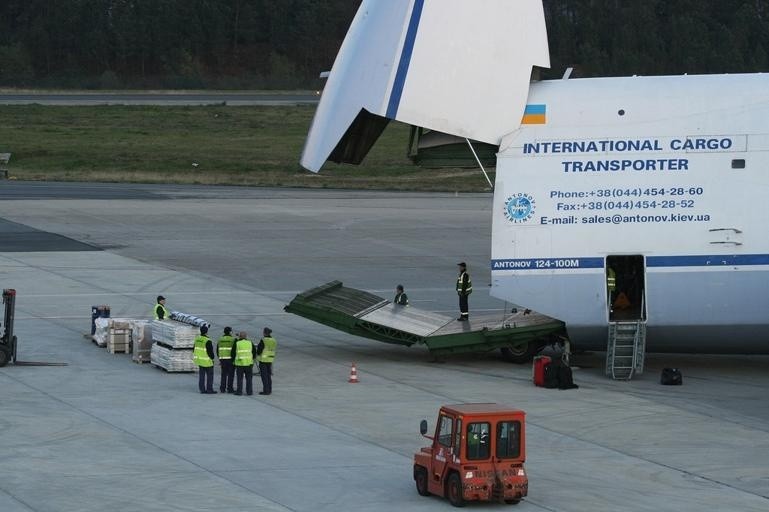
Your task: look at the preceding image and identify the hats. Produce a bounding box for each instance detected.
[157,296,165,301]
[457,262,466,267]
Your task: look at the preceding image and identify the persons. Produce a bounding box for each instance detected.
[153,296,167,321]
[193,325,277,395]
[394,285,408,305]
[608,268,616,291]
[537,337,570,361]
[455,262,472,321]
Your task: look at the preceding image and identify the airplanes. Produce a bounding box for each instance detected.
[282,0,768,380]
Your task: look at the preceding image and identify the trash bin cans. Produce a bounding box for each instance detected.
[91,305,109,347]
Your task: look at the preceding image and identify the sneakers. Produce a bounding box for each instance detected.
[456,315,468,320]
[200,390,272,396]
